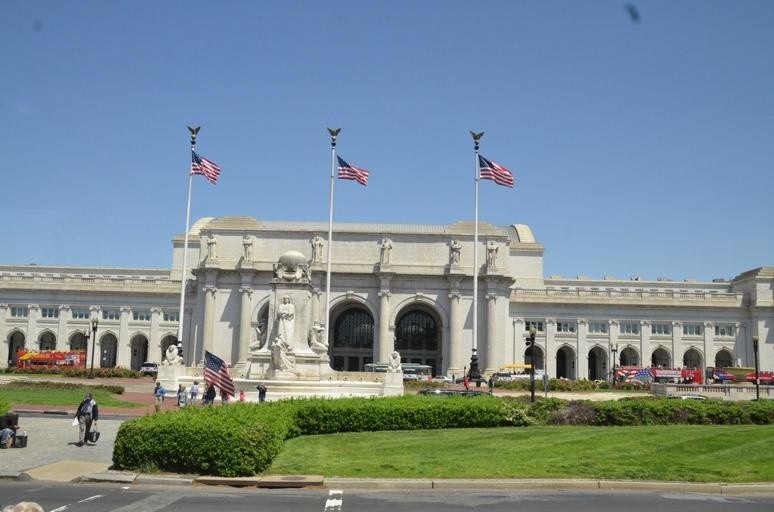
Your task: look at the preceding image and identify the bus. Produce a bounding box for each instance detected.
[364,361,433,383]
[14,347,88,375]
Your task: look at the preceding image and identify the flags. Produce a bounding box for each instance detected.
[477,152,513,188]
[203,351,234,397]
[615,366,655,382]
[336,154,369,186]
[190,148,220,187]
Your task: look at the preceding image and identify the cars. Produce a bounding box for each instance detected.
[432,374,453,384]
[454,376,467,384]
[591,379,646,386]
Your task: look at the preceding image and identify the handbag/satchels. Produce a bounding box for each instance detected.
[12,435,27,447]
[89,432,99,442]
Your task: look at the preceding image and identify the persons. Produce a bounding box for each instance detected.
[255,384,266,402]
[487,377,492,393]
[377,236,393,264]
[72,392,100,447]
[151,381,165,413]
[276,294,295,346]
[692,364,701,384]
[205,231,218,259]
[175,379,232,408]
[485,240,499,269]
[239,389,244,401]
[242,232,253,260]
[311,234,324,263]
[0,410,21,449]
[682,364,689,382]
[450,238,463,265]
[463,374,470,389]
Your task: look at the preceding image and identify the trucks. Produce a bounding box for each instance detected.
[613,363,738,389]
[743,371,774,386]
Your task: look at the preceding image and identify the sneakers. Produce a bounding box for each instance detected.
[0,444,7,449]
[79,440,83,446]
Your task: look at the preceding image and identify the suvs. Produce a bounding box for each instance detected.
[139,362,158,376]
[416,387,497,399]
[666,393,709,401]
[489,371,512,385]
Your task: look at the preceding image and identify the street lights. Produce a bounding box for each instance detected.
[525,321,537,404]
[751,334,762,400]
[608,342,620,383]
[87,316,99,379]
